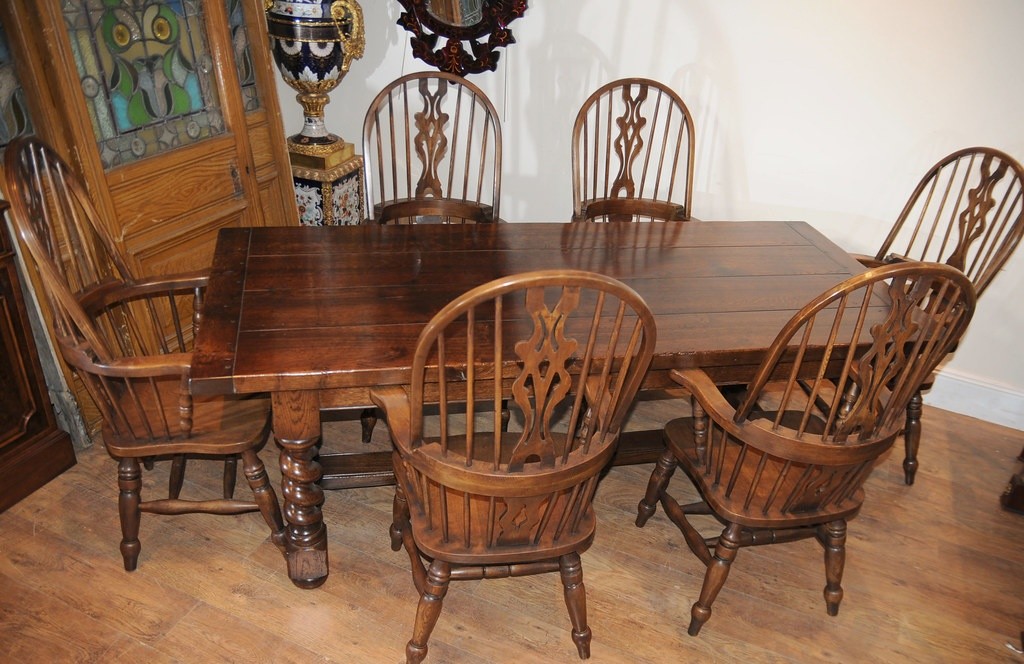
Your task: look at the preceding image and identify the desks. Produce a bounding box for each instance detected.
[190,222,957,592]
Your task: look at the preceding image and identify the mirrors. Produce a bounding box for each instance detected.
[396,0,528,84]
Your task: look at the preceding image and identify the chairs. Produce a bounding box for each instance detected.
[634,260,977,635]
[792,147,1024,482]
[369,267,657,663]
[360,73,504,225]
[2,135,288,572]
[572,77,702,223]
